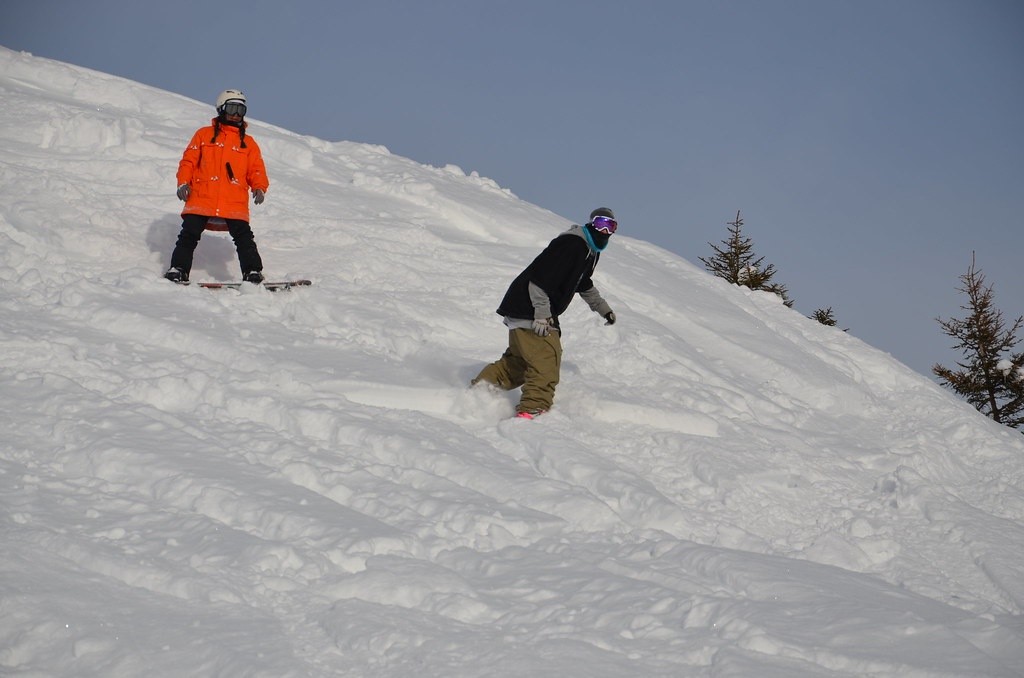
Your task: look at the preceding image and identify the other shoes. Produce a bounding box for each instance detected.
[517,411,541,420]
[243,272,263,284]
[166,264,189,283]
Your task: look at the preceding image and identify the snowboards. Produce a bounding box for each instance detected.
[172,278,314,293]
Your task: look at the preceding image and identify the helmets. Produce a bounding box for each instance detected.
[216,89,246,115]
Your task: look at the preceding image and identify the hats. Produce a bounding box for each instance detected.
[591,207,614,220]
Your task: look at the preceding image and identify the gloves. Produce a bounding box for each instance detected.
[604,311,616,326]
[177,183,192,201]
[532,316,554,337]
[253,189,265,205]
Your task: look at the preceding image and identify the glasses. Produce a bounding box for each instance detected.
[592,216,617,235]
[222,103,247,117]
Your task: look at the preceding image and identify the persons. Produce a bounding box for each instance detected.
[466,207,618,420]
[163,89,274,287]
